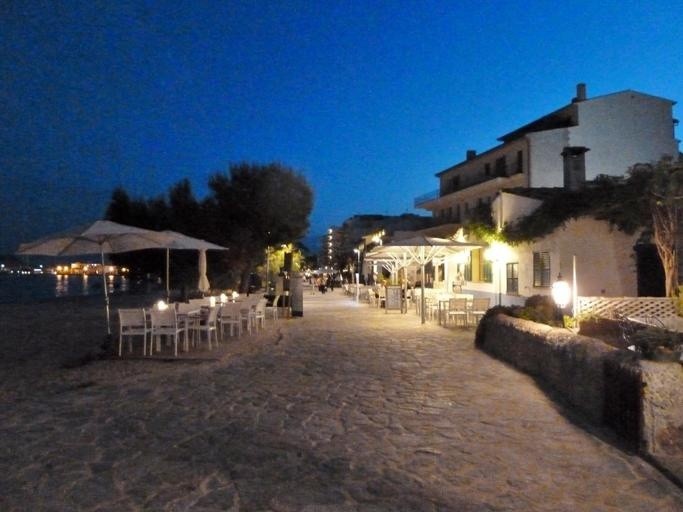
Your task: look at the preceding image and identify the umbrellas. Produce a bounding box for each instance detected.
[156,230,230,305]
[197,248,211,299]
[14,220,183,335]
[362,230,490,324]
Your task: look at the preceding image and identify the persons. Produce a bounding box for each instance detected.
[309,272,348,295]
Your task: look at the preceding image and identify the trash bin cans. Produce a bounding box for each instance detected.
[386,286,402,309]
[289,277,303,316]
[451,281,462,293]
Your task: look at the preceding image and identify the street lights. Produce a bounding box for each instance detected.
[352,248,363,305]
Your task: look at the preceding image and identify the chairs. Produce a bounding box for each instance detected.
[344,283,491,330]
[117,292,280,357]
